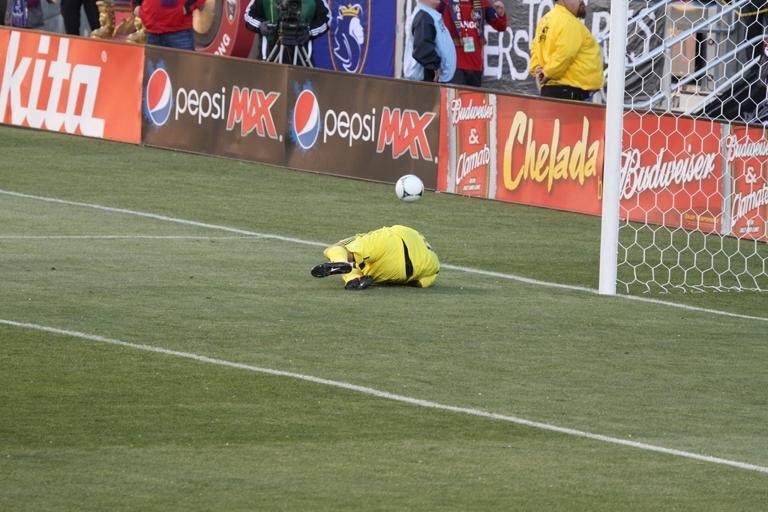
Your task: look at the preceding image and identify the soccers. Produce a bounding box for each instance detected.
[395,174,424,203]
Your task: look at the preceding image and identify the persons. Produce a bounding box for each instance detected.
[133,0,206,51]
[528,0,604,101]
[311,224,440,289]
[244,0,333,68]
[403,0,457,84]
[47,0,101,36]
[436,0,507,87]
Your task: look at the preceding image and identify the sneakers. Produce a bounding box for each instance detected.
[311,262,353,277]
[345,274,375,290]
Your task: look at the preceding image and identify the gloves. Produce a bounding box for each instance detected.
[260,20,272,35]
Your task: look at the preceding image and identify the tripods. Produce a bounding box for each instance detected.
[265,36,314,68]
